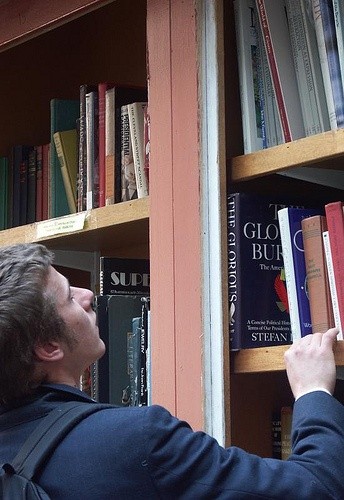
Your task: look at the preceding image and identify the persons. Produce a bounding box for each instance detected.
[0,242,343,500]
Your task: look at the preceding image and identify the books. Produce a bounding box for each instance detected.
[225,188,344,351]
[271,405,295,460]
[1,82,151,229]
[231,0,344,154]
[76,256,151,407]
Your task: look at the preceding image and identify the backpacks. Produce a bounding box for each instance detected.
[0,401,118,500]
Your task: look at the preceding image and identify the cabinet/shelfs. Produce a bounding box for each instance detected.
[0,0,176,420]
[169,0,344,456]
